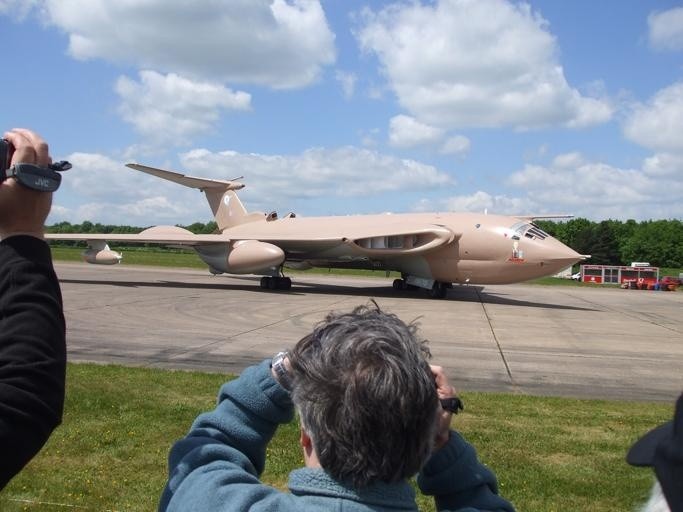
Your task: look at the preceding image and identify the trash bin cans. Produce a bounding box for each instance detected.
[630,280,636,289]
[654,283,659,290]
[642,284,648,290]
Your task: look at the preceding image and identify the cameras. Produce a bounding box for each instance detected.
[0,139,16,184]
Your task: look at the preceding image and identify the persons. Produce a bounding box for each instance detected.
[156,294,514,510]
[0,124,66,497]
[625,394,682,512]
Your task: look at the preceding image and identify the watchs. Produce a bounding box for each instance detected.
[270,348,295,393]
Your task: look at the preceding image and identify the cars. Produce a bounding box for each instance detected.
[571,272,581,281]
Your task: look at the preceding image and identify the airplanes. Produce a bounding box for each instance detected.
[43,162,592,299]
